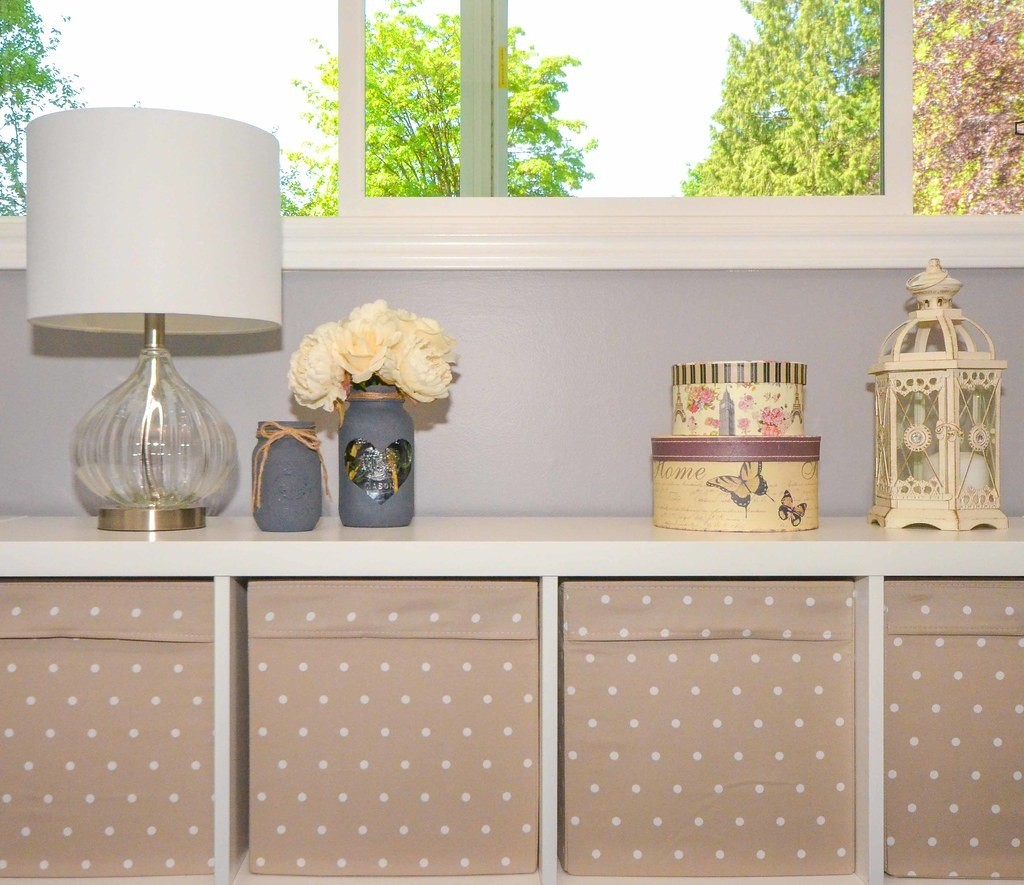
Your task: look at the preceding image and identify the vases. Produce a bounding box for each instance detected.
[335,379,416,528]
[251,421,331,532]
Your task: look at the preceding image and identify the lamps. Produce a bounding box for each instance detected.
[25,108,287,533]
[869,257,1005,535]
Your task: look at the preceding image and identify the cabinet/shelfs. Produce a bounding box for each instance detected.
[0,514,1024,884]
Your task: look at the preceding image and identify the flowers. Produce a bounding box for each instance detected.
[287,299,458,414]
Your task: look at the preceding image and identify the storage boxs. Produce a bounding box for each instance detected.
[651,434,822,532]
[671,358,806,436]
